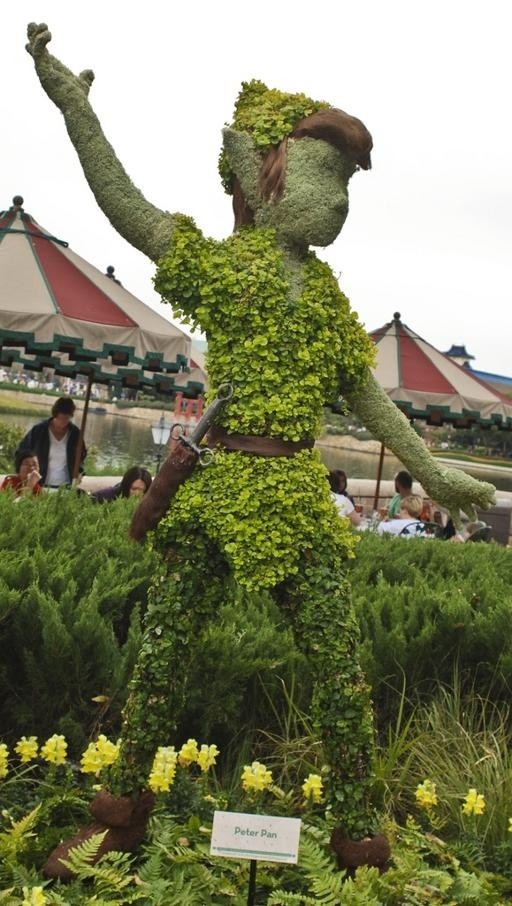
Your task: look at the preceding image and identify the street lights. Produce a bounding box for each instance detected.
[150,411,173,474]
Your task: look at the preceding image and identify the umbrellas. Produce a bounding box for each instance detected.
[0,266,209,489]
[323,312,512,509]
[0,196,192,373]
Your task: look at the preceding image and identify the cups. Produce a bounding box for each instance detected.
[381,508,388,523]
[354,504,365,512]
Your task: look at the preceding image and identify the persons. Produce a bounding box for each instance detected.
[20,398,87,490]
[90,467,152,505]
[0,449,43,497]
[325,469,478,539]
[25,22,495,886]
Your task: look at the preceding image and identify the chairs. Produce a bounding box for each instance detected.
[398,517,492,543]
